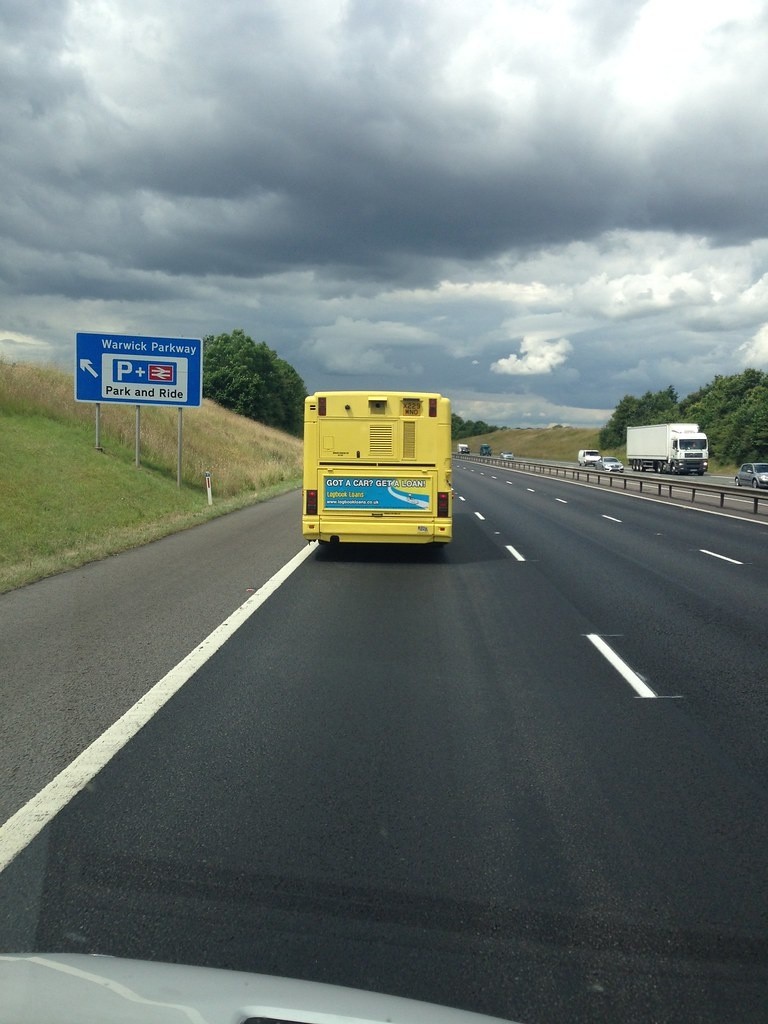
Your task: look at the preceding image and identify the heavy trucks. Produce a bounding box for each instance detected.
[626,422,709,477]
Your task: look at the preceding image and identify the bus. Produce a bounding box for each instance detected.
[300,390,453,552]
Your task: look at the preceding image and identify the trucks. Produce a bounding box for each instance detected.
[481,444,492,456]
[578,449,602,466]
[457,444,470,454]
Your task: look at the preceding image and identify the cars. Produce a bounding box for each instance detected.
[500,451,515,461]
[734,461,768,488]
[594,455,625,474]
